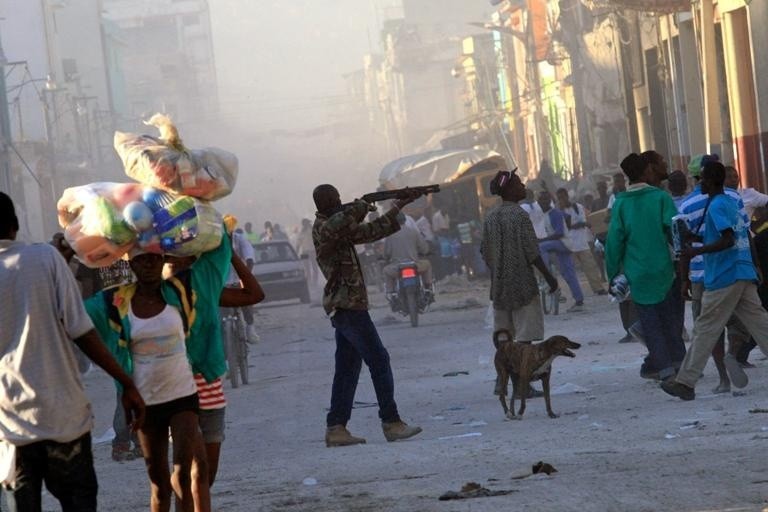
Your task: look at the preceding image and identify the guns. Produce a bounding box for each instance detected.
[359,183,441,221]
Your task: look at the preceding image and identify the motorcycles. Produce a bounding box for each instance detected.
[386,261,434,328]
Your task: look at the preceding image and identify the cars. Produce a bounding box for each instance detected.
[246,243,310,305]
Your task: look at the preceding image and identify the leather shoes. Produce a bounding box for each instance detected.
[640,367,658,378]
[660,380,695,400]
[325,425,366,448]
[619,334,637,343]
[382,422,422,441]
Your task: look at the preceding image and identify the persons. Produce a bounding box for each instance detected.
[357,205,477,302]
[479,168,558,399]
[619,150,767,401]
[313,183,424,448]
[0,191,148,512]
[243,216,319,282]
[47,212,266,512]
[521,172,626,310]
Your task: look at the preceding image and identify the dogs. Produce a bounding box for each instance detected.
[491,327,583,421]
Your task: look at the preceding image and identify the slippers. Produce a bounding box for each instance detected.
[712,386,729,394]
[722,353,749,388]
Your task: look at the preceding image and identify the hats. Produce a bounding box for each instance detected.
[687,153,719,179]
[489,166,518,196]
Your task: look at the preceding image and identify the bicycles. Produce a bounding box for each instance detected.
[220,306,253,389]
[539,257,566,317]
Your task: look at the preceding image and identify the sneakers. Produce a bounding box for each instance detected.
[566,304,584,312]
[246,329,261,344]
